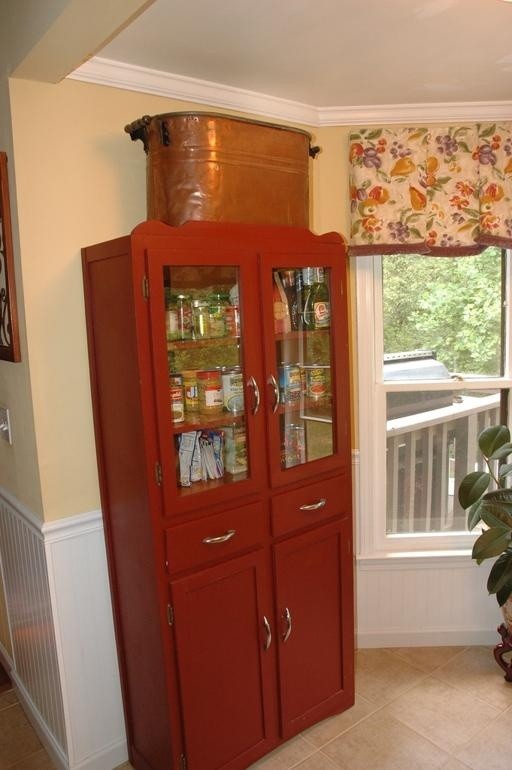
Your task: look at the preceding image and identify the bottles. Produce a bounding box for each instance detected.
[163,291,241,343]
[169,368,224,423]
[273,267,332,331]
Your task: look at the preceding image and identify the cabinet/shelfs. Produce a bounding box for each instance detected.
[81,219,355,770]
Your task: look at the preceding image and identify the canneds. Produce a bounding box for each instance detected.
[163,292,241,342]
[278,360,327,405]
[167,364,245,424]
[279,423,307,469]
